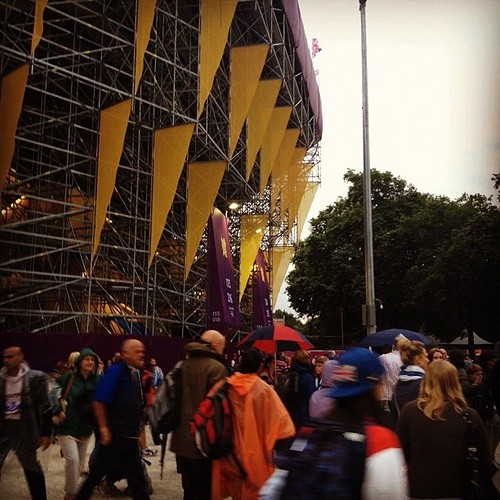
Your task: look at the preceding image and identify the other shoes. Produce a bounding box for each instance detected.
[79,470,89,478]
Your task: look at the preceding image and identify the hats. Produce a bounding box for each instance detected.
[322,348,383,397]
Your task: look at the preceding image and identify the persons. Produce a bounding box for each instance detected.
[48,341,499,434]
[49,346,106,499]
[162,329,232,500]
[255,346,412,500]
[0,346,55,500]
[393,359,491,500]
[72,338,155,499]
[190,346,297,499]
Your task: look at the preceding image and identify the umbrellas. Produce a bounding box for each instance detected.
[356,328,434,349]
[236,325,316,354]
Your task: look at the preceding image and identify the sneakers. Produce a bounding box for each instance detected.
[139,447,157,456]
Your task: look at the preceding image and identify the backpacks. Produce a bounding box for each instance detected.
[272,416,376,500]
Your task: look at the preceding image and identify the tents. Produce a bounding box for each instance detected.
[448,328,495,359]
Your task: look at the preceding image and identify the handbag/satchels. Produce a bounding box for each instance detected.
[61,374,74,412]
[194,379,233,459]
[463,410,490,500]
[155,368,183,435]
